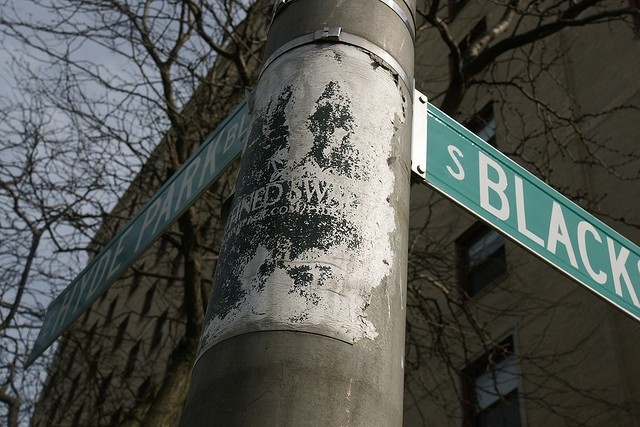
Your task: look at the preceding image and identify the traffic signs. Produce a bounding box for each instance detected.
[21,82,251,372]
[412,87,640,322]
